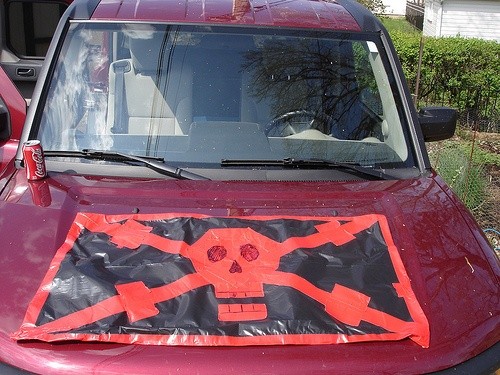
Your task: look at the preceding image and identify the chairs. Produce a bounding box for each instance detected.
[104,31,195,135]
[237,38,326,137]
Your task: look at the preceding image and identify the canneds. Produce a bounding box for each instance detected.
[21,139,48,181]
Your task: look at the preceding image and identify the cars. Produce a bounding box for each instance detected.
[0,0,500,375]
